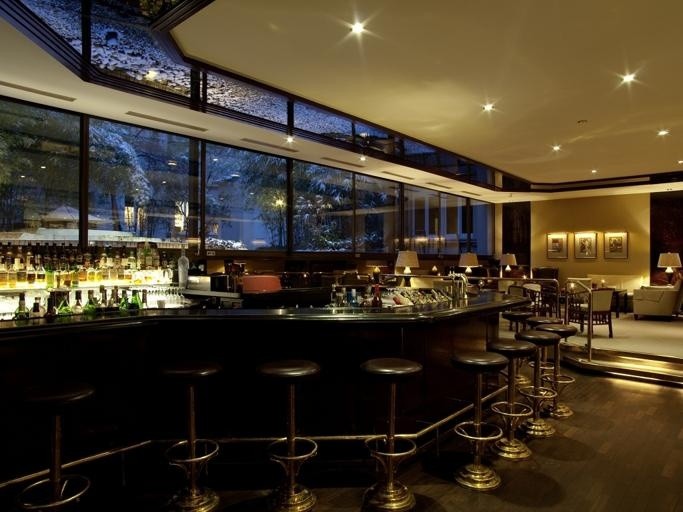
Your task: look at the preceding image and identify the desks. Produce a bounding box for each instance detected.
[542,289,589,333]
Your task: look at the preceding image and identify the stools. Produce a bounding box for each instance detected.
[502,311,534,386]
[453,352,509,491]
[515,330,560,439]
[526,317,561,386]
[163,363,222,511]
[19,386,94,511]
[487,340,537,461]
[512,312,533,361]
[362,357,423,511]
[259,362,321,511]
[536,324,577,418]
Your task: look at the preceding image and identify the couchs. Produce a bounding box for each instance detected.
[673,287,683,317]
[587,274,643,313]
[568,278,591,312]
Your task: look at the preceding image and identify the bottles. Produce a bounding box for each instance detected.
[349,288,357,312]
[34,243,41,266]
[15,300,28,319]
[51,242,176,290]
[109,289,117,307]
[57,293,70,314]
[118,290,129,316]
[26,257,35,289]
[19,292,26,301]
[141,290,149,308]
[36,259,46,288]
[113,285,120,299]
[46,262,54,288]
[16,259,26,289]
[4,241,14,262]
[14,245,25,270]
[24,240,33,269]
[0,242,4,257]
[99,285,104,294]
[83,290,94,309]
[0,258,7,289]
[129,289,139,316]
[371,284,382,310]
[71,291,82,313]
[30,302,40,316]
[28,297,44,316]
[137,291,141,310]
[177,245,189,288]
[97,290,108,308]
[43,242,49,267]
[44,298,55,317]
[7,257,16,288]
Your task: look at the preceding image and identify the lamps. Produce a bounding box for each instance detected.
[657,252,682,287]
[396,250,420,287]
[459,253,478,276]
[366,260,388,284]
[500,254,517,277]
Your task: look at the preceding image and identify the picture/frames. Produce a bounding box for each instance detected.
[574,231,597,259]
[547,232,568,259]
[604,232,628,259]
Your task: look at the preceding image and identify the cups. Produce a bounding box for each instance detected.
[146,287,186,307]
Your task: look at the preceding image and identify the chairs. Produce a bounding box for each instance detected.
[532,268,559,317]
[503,285,525,331]
[530,279,559,317]
[498,279,515,292]
[565,288,616,362]
[634,280,681,321]
